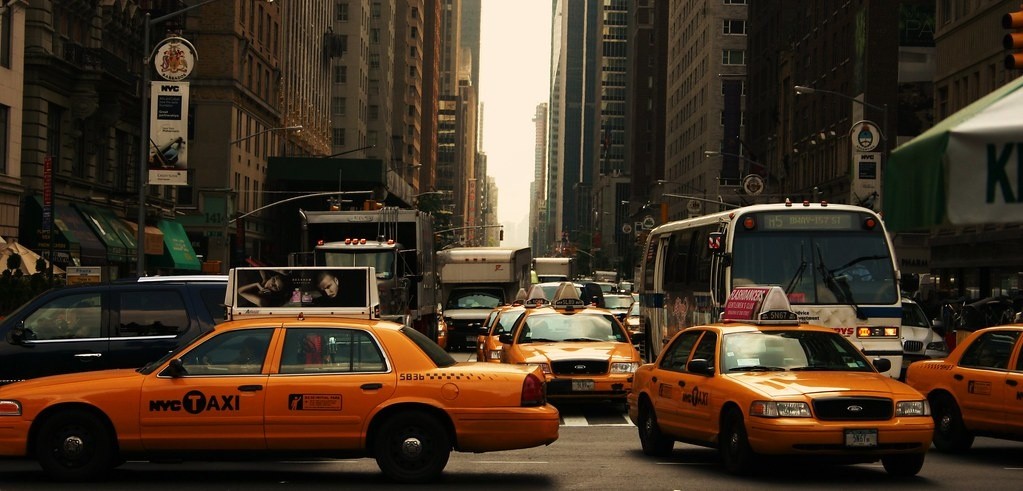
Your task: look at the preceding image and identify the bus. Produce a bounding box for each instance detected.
[637,198,918,380]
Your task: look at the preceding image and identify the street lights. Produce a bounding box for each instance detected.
[704,151,782,203]
[795,85,888,173]
[223,124,304,276]
[659,180,706,215]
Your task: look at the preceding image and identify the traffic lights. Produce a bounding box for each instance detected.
[1001,5,1023,75]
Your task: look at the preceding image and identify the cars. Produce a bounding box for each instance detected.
[906,323,1023,458]
[499,281,647,414]
[901,297,949,379]
[623,301,641,345]
[626,285,937,479]
[438,303,452,350]
[525,277,639,302]
[602,294,635,324]
[0,266,560,491]
[476,288,526,362]
[483,284,552,363]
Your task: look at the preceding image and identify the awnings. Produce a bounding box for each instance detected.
[18,197,201,271]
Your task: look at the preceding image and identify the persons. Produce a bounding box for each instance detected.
[206,336,264,374]
[237,269,349,307]
[43,303,96,338]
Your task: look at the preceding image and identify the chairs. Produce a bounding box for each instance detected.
[727,350,784,368]
[531,321,550,339]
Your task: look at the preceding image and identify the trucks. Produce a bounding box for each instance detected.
[437,247,532,352]
[299,207,438,343]
[531,256,572,282]
